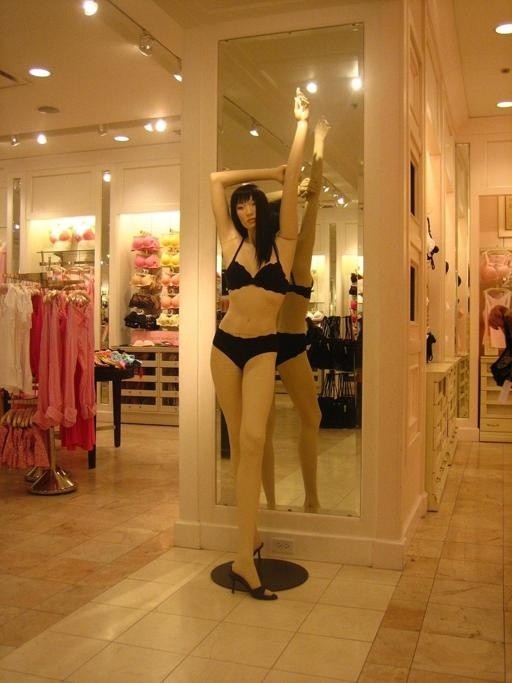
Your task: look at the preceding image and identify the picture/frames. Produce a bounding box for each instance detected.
[494,192,512,241]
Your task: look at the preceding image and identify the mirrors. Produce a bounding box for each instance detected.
[453,137,473,420]
[205,18,382,528]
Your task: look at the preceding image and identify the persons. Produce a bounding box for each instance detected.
[205,85,312,602]
[260,114,334,513]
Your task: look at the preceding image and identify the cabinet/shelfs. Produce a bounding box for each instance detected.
[271,258,327,391]
[476,351,511,444]
[424,348,469,516]
[111,345,182,428]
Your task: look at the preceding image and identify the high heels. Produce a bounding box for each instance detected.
[253,542,264,563]
[232,572,278,602]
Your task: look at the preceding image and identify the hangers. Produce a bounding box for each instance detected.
[481,242,510,258]
[2,265,92,310]
[131,225,179,317]
[0,392,42,433]
[481,282,510,299]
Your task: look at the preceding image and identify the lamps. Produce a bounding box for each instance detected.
[6,114,172,146]
[135,27,182,83]
[243,113,355,212]
